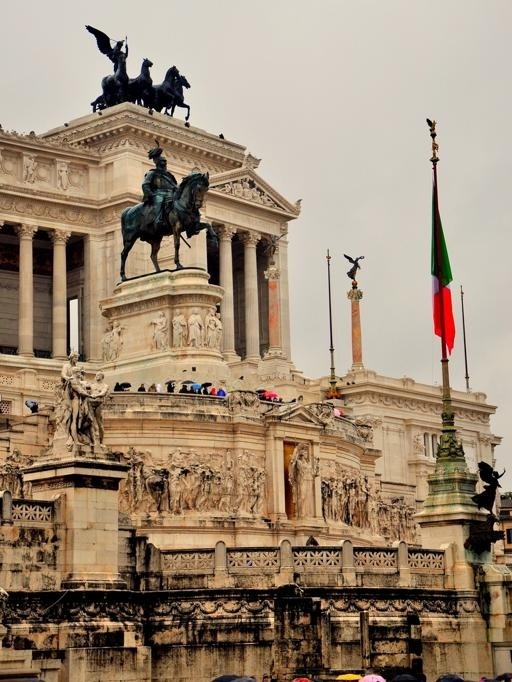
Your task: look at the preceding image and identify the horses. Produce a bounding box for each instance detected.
[100,52,189,120]
[118,171,218,281]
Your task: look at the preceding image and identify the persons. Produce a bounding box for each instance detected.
[150,307,224,351]
[59,162,71,190]
[26,155,35,182]
[296,450,319,517]
[471,461,506,514]
[142,139,177,230]
[85,24,128,72]
[115,379,229,396]
[61,350,107,446]
[347,257,361,282]
[259,392,296,403]
[30,402,38,413]
[108,320,123,359]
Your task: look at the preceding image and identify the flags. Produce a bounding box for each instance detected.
[430,166,455,353]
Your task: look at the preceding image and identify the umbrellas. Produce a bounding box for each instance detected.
[212,673,511,682]
[256,389,266,393]
